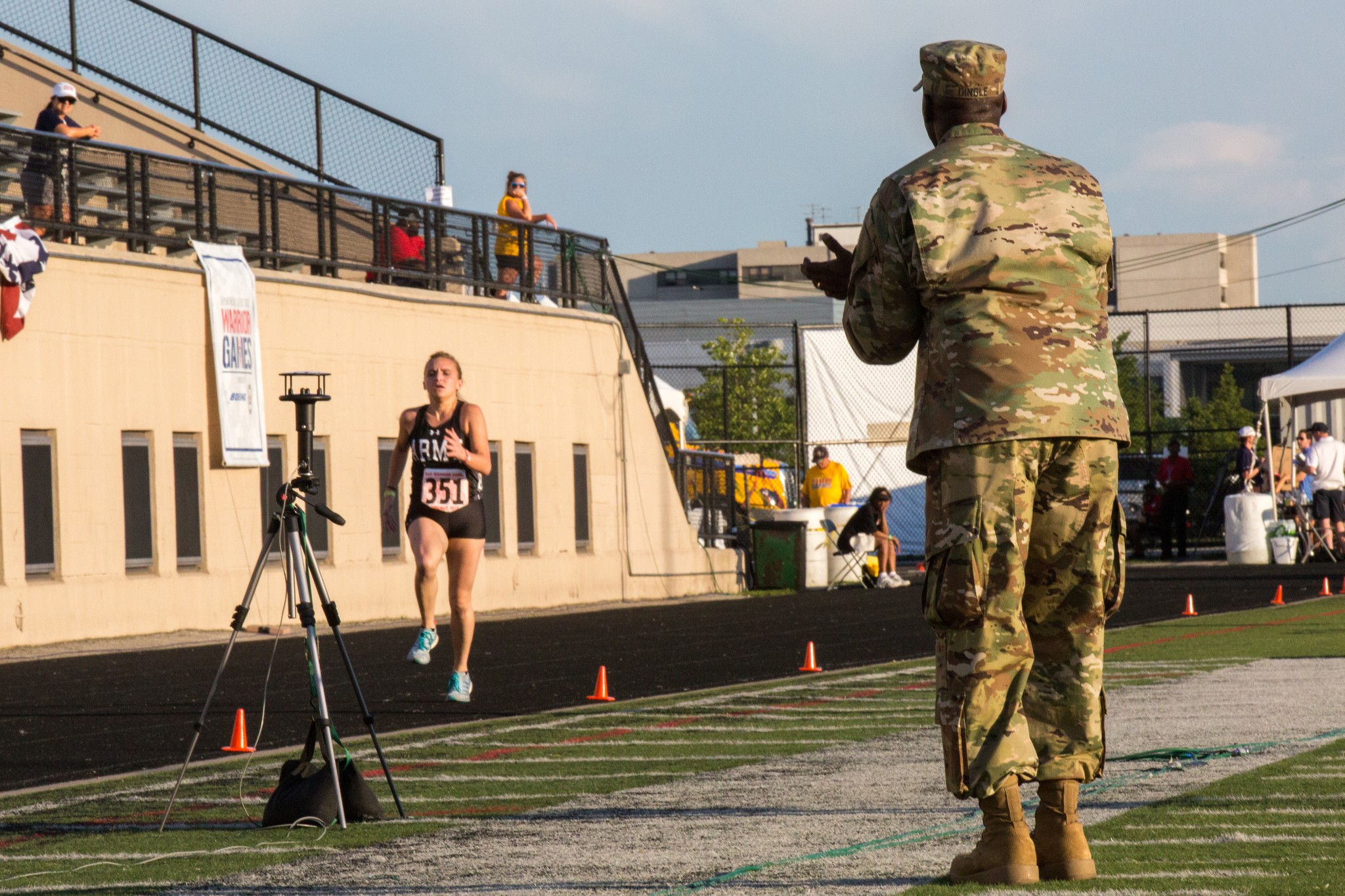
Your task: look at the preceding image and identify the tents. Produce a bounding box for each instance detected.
[1260,330,1345,523]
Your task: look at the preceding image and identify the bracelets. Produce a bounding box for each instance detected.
[384,492,398,498]
[463,448,472,463]
[889,534,892,540]
[522,196,527,199]
[385,486,398,492]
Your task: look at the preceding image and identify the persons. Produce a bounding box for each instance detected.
[1136,437,1192,561]
[837,486,910,589]
[800,37,1135,887]
[363,207,434,287]
[381,351,492,703]
[1284,423,1344,559]
[493,172,558,307]
[1236,427,1268,492]
[802,446,853,508]
[20,81,100,245]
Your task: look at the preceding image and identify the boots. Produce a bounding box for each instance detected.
[1031,780,1099,880]
[949,774,1041,884]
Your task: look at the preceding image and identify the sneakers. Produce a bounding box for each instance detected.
[447,669,473,702]
[407,619,440,665]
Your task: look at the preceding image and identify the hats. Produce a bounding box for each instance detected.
[913,40,1007,97]
[52,82,77,100]
[1307,422,1328,431]
[1238,425,1261,438]
[812,446,828,463]
[398,207,423,222]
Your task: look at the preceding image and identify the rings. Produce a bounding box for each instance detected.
[817,282,821,287]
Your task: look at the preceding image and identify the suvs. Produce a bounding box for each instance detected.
[1114,453,1165,548]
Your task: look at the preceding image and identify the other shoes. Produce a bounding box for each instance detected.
[1177,550,1187,558]
[1161,550,1171,559]
[877,574,911,589]
[524,296,541,305]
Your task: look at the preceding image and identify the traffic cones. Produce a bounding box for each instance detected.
[798,642,821,671]
[220,708,256,753]
[1340,576,1345,594]
[1271,584,1285,604]
[587,666,615,701]
[1319,577,1333,596]
[1182,593,1197,616]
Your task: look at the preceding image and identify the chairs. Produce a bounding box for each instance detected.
[820,519,877,592]
[1284,491,1338,566]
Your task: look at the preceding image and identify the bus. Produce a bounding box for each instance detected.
[652,409,797,538]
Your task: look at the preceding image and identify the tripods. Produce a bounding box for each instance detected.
[155,483,405,833]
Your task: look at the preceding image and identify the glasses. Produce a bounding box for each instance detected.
[1296,437,1304,440]
[57,97,76,104]
[511,182,525,188]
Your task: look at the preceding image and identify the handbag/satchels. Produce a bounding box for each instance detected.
[335,756,385,823]
[261,758,341,827]
